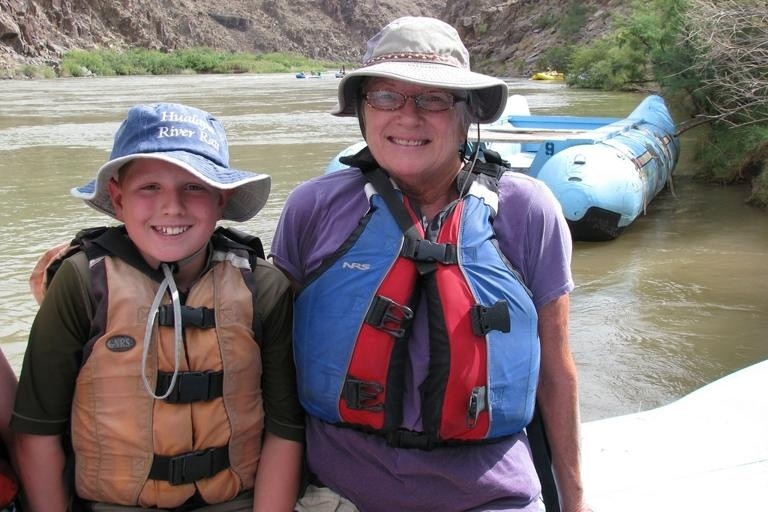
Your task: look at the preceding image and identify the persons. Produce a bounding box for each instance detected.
[29,15,594,512]
[7,103,306,512]
[0,348,27,512]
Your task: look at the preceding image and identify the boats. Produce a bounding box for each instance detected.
[323,93,681,244]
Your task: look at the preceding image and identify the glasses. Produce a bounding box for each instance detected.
[362,89,466,111]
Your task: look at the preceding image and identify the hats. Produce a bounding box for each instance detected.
[330,15,508,124]
[70,102,271,222]
